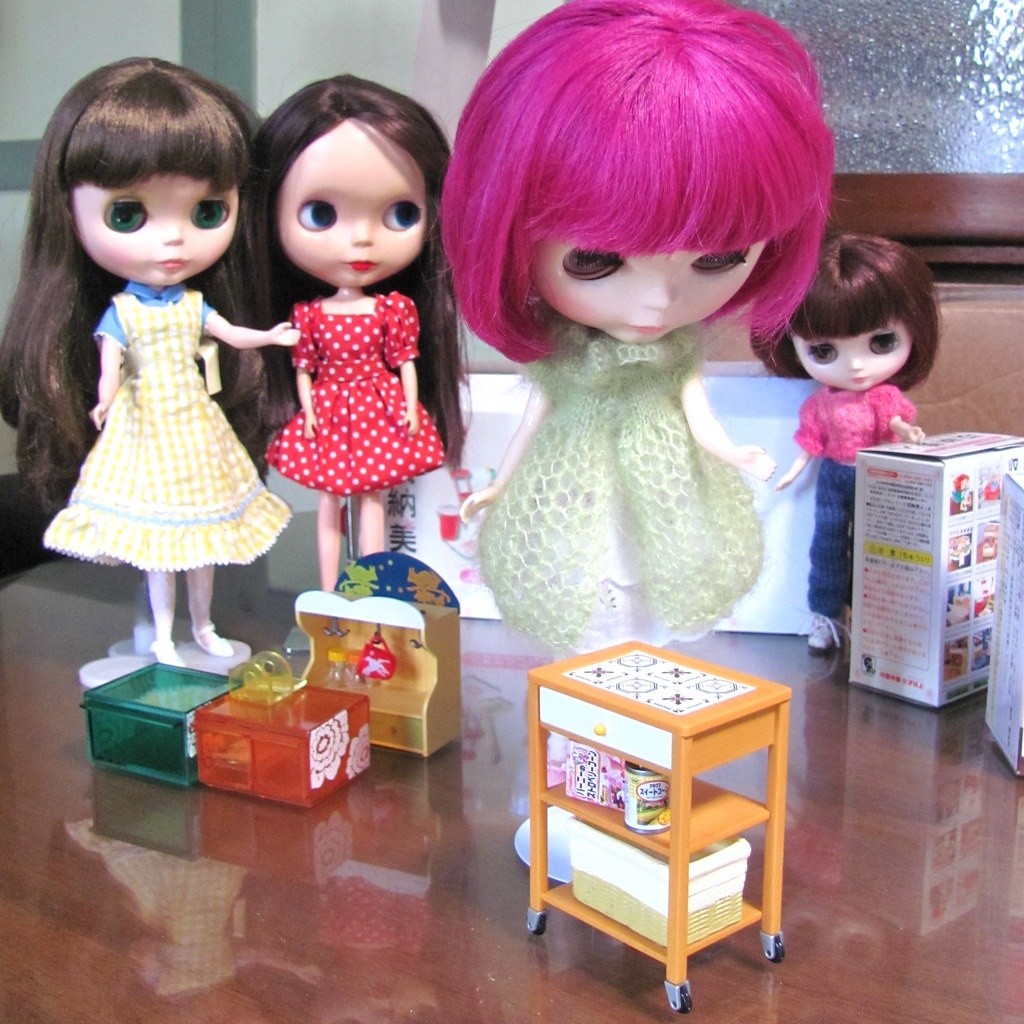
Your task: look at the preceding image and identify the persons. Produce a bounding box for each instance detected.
[445,0,834,678]
[241,74,465,596]
[0,55,299,665]
[748,232,941,650]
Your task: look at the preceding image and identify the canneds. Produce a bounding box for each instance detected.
[625,760,672,834]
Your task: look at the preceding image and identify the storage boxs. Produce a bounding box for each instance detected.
[846,433,1024,707]
[383,361,823,635]
[981,468,1024,778]
[81,663,244,787]
[569,816,753,948]
[191,679,371,808]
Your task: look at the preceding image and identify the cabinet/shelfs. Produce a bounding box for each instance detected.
[293,589,461,759]
[527,638,793,1012]
